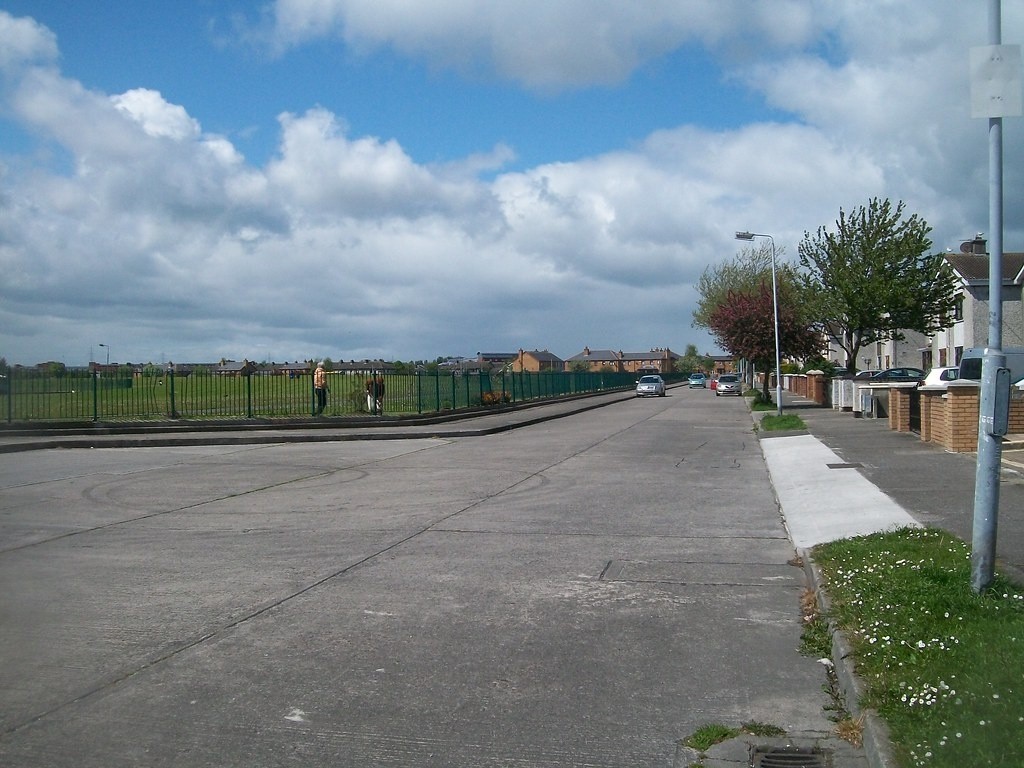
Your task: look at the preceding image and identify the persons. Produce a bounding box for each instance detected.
[365,377,385,411]
[314,362,330,415]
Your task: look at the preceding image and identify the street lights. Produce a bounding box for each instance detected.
[98,344,110,376]
[734,231,783,417]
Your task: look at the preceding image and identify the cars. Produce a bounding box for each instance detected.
[714,375,744,397]
[873,367,927,382]
[636,376,666,397]
[921,367,961,386]
[856,370,913,378]
[688,373,707,389]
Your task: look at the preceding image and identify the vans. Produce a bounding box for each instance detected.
[957,347,1024,385]
